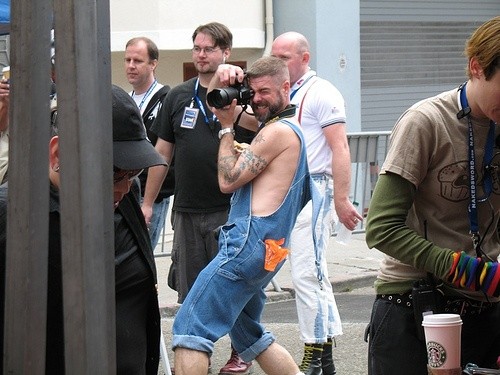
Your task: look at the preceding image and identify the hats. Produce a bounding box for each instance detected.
[51,84,168,170]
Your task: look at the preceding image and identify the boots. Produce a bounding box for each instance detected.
[299,342,324,375]
[322,337,336,375]
[218,343,256,375]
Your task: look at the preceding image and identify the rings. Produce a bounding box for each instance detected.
[354,218,357,221]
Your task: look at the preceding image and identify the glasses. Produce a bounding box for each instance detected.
[113,166,145,182]
[190,46,223,55]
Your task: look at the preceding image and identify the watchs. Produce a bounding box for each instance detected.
[219,128,236,139]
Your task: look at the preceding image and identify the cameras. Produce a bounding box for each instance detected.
[206,73,255,109]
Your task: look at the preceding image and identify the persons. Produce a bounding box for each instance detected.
[141,21,254,375]
[365,16,500,375]
[271,31,363,375]
[125,37,171,252]
[172,55,305,375]
[0,30,169,375]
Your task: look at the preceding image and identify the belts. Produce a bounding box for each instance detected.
[377,290,500,322]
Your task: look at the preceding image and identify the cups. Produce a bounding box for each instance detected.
[2,65,12,85]
[421,314,464,369]
